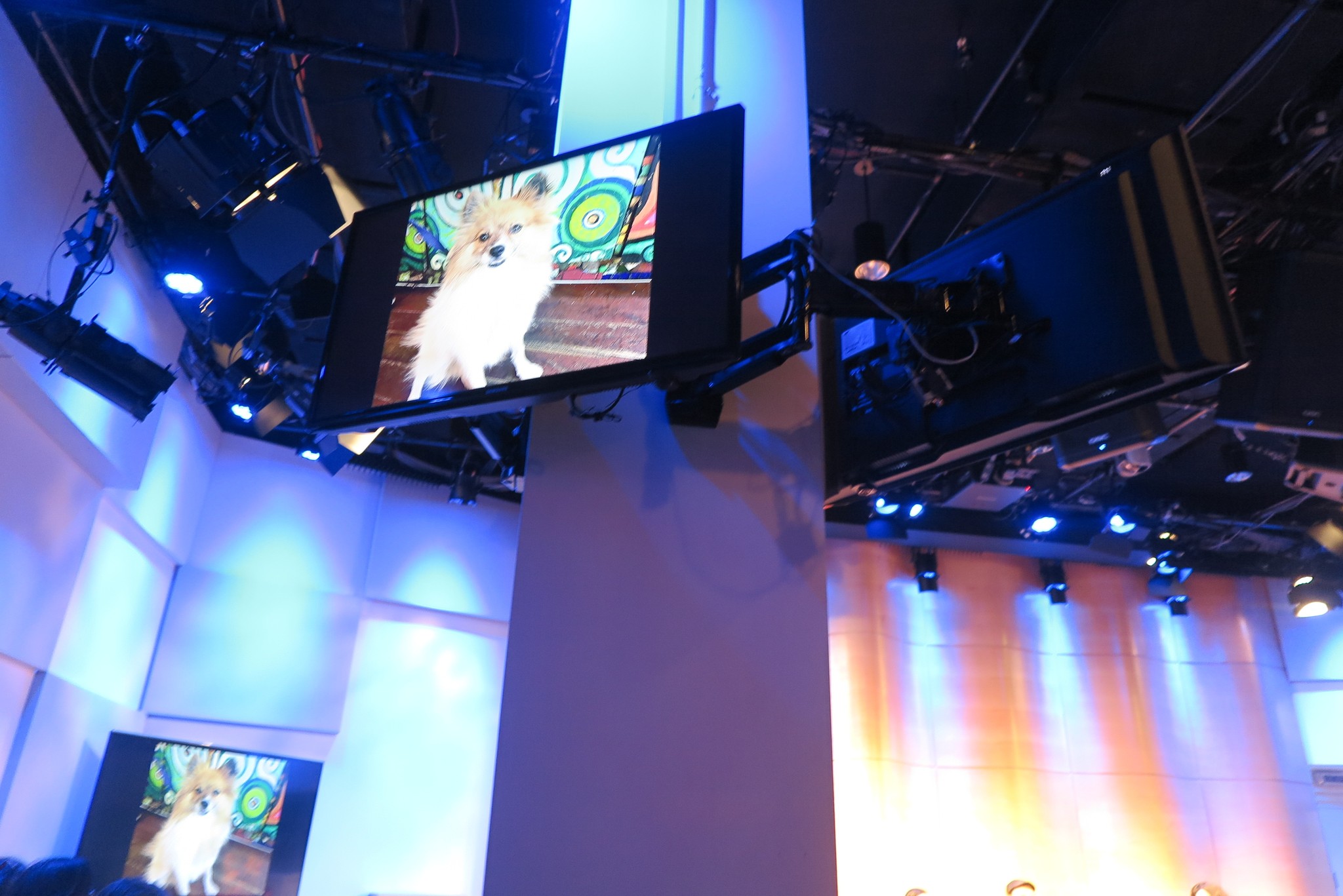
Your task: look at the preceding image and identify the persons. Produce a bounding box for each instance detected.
[0,854,167,896]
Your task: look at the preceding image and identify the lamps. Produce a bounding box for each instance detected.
[872,481,1329,618]
[86,15,482,508]
[850,159,891,281]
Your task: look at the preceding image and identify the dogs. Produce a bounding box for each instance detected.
[401,173,562,403]
[139,752,241,895]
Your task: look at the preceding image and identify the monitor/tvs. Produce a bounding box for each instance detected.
[820,120,1252,513]
[309,104,743,436]
[72,730,323,896]
